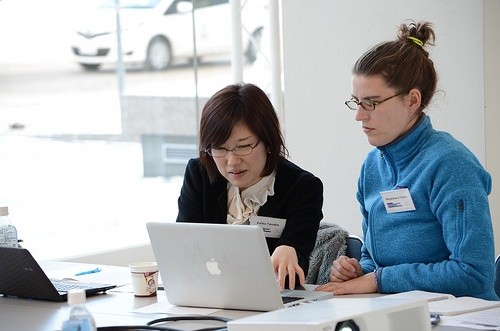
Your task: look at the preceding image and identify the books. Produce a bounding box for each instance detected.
[375,290,500,315]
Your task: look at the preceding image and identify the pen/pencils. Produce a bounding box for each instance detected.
[74,268,103,277]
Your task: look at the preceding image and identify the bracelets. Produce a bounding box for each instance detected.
[374,269,380,293]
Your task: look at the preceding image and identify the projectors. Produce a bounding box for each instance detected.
[227,293,434,331]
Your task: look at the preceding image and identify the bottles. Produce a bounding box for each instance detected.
[57,288,98,331]
[0,206,19,248]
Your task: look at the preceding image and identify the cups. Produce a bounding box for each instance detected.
[128,262,159,297]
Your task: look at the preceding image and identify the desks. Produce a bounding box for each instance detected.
[0,260,500,331]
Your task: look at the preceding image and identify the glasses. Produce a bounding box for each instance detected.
[344,87,413,111]
[204,138,261,158]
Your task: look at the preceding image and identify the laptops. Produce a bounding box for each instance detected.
[146,221,334,313]
[0,248,117,300]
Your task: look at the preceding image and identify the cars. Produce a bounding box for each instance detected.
[68,0,270,75]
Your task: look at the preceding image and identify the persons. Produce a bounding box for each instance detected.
[176,83,324,292]
[315,19,500,301]
